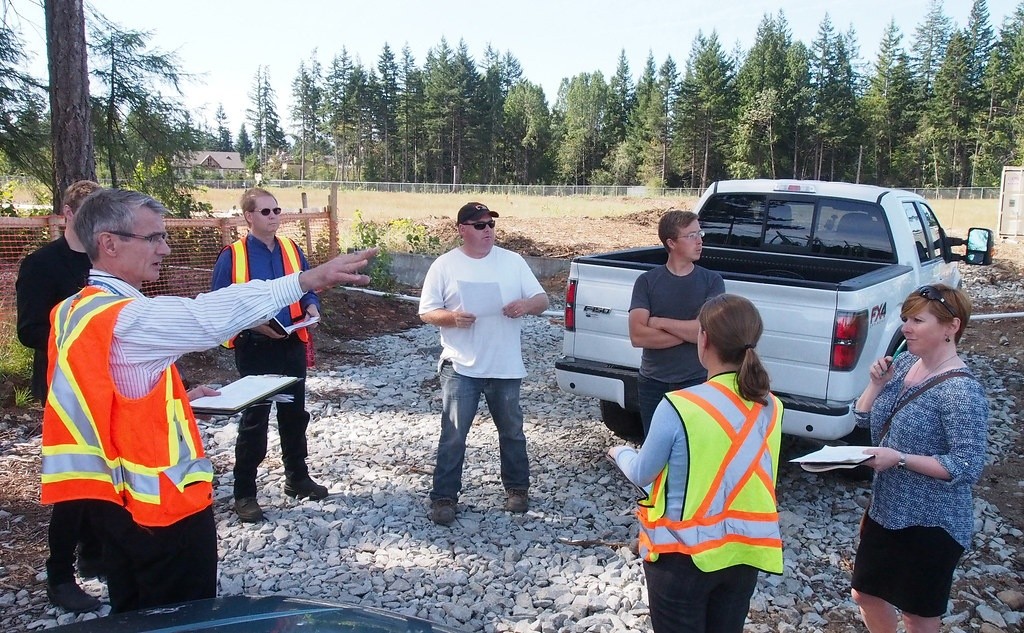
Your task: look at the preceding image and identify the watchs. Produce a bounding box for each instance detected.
[896,451,906,471]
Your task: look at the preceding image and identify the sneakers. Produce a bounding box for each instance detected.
[508,487,529,513]
[284,470,328,500]
[234,492,263,523]
[430,496,458,524]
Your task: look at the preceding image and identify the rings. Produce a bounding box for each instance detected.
[515,311,518,314]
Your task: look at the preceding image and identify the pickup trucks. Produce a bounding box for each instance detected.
[555,178,993,443]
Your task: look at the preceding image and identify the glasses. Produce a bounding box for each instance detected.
[248,207,282,216]
[461,219,496,231]
[919,285,958,320]
[96,230,169,247]
[674,229,705,240]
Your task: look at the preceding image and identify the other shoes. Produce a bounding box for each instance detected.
[77,563,107,577]
[46,577,102,612]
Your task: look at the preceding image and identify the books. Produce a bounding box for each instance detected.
[269,317,319,336]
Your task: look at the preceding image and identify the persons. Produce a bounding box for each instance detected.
[41,188,380,619]
[628,211,726,439]
[211,188,328,522]
[419,202,550,525]
[608,292,784,633]
[851,284,988,633]
[16,182,111,612]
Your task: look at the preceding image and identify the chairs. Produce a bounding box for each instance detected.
[835,212,875,236]
[768,203,791,226]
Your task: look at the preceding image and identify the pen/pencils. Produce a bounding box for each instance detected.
[302,308,321,326]
[881,339,907,376]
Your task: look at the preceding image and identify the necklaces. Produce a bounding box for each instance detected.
[906,354,957,386]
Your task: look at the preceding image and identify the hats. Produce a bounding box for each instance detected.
[458,201,499,223]
